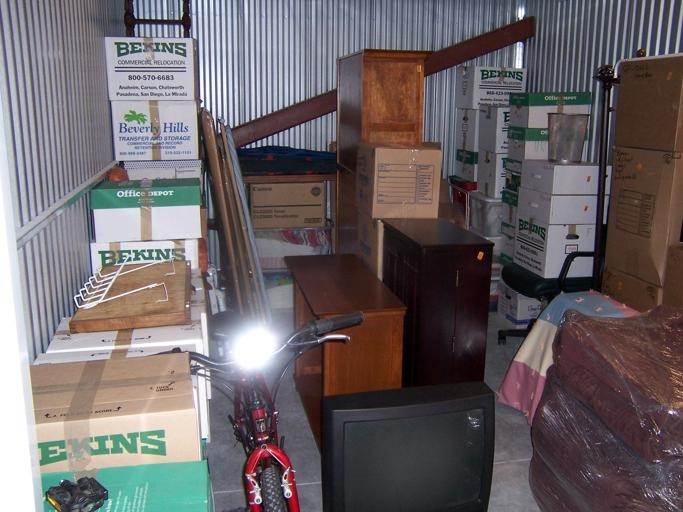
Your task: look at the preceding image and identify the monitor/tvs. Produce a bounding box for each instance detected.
[319,378,497,512]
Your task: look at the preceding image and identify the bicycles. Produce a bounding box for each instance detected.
[141,305,364,511]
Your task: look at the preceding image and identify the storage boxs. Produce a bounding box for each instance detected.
[28,34,216,512]
[251,183,326,228]
[453,53,682,326]
[355,139,441,281]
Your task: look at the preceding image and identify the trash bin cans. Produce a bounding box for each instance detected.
[548,113,591,164]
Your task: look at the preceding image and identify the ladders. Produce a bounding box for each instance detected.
[123,3,191,36]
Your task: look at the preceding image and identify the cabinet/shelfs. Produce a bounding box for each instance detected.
[335,48,429,250]
[382,220,493,388]
[240,170,337,312]
[284,252,407,458]
